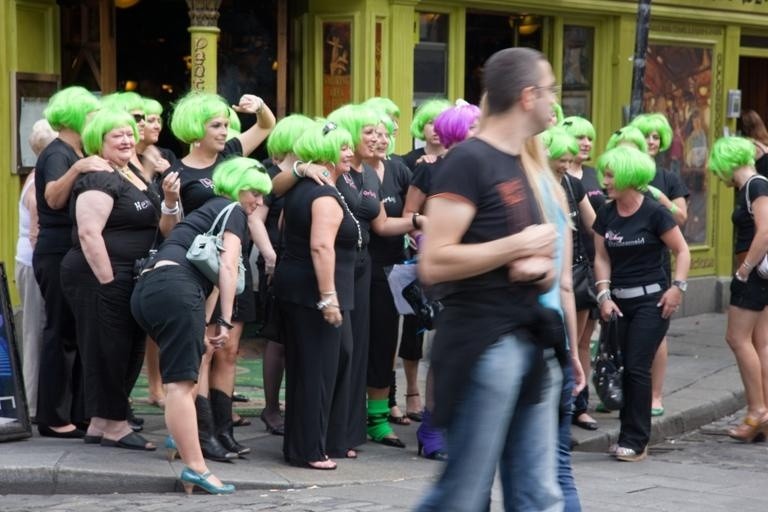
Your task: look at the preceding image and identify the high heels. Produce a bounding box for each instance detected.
[728,411,768,443]
[181,467,236,495]
[164,435,181,462]
[261,409,285,436]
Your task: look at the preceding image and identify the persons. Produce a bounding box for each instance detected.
[411,48,590,512]
[14,84,768,496]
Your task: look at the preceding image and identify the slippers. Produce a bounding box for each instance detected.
[83,435,100,444]
[98,435,156,451]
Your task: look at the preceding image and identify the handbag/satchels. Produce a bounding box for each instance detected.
[383,260,419,315]
[571,255,599,312]
[402,277,443,330]
[186,203,247,297]
[755,252,768,278]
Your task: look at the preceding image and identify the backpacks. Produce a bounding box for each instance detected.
[592,340,626,411]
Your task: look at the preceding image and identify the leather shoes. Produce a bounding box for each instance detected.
[651,407,665,416]
[127,394,166,432]
[307,389,448,471]
[38,424,85,439]
[232,387,251,428]
[573,412,600,431]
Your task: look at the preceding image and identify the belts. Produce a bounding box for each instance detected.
[611,284,662,300]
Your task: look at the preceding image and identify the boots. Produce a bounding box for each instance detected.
[196,394,238,462]
[211,388,252,455]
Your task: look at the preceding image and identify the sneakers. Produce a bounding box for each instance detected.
[615,446,648,462]
[609,443,620,455]
